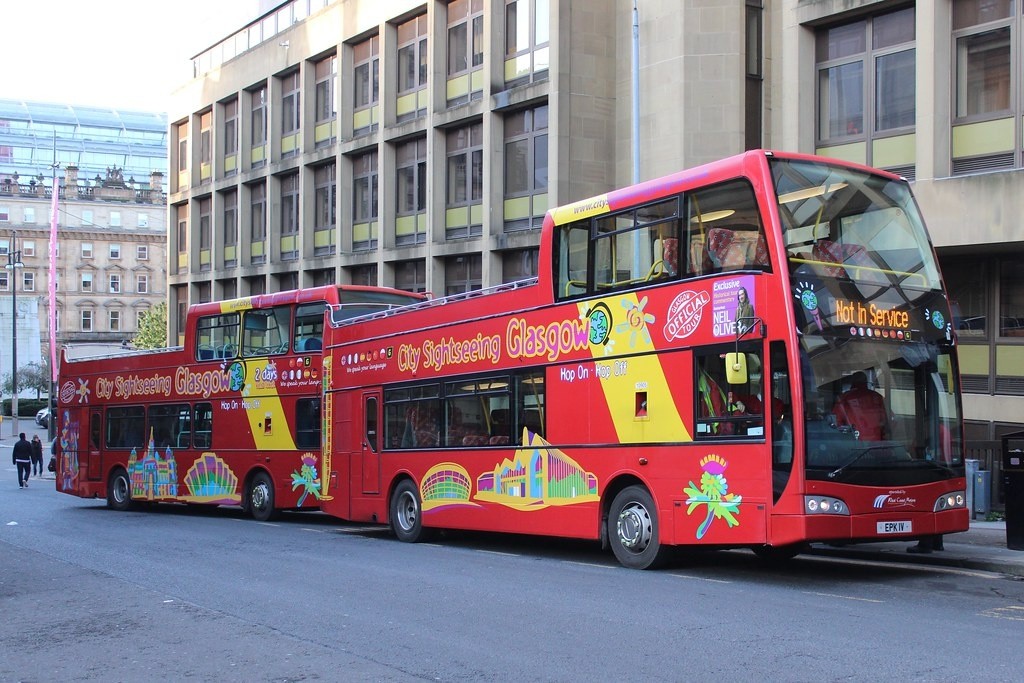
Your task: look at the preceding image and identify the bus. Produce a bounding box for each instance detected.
[319,148,970,568]
[56,282,440,523]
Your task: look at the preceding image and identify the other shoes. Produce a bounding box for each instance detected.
[20,486,24,489]
[23,480,28,488]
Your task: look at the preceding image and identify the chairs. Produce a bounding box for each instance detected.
[160,439,174,447]
[661,229,876,283]
[392,406,510,448]
[125,438,142,447]
[199,338,322,361]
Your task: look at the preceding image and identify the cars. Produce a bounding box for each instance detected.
[36,405,49,428]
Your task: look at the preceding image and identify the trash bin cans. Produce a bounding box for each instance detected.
[1002,430,1024,551]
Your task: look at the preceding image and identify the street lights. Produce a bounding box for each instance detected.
[5,230,25,438]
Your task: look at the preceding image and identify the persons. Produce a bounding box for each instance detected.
[51,430,58,458]
[831,371,889,441]
[30,435,44,478]
[907,412,952,554]
[734,286,754,333]
[12,433,33,489]
[747,375,783,428]
